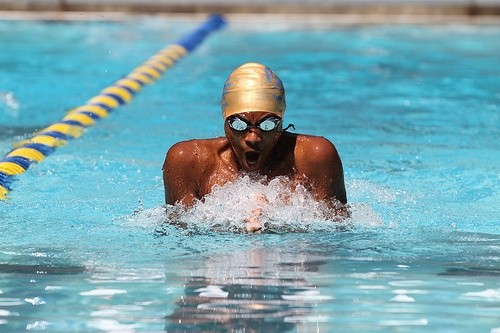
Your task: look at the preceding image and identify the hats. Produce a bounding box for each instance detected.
[223,62,286,117]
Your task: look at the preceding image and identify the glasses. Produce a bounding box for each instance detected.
[229,114,281,131]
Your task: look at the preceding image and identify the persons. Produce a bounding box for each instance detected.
[160,62,351,229]
